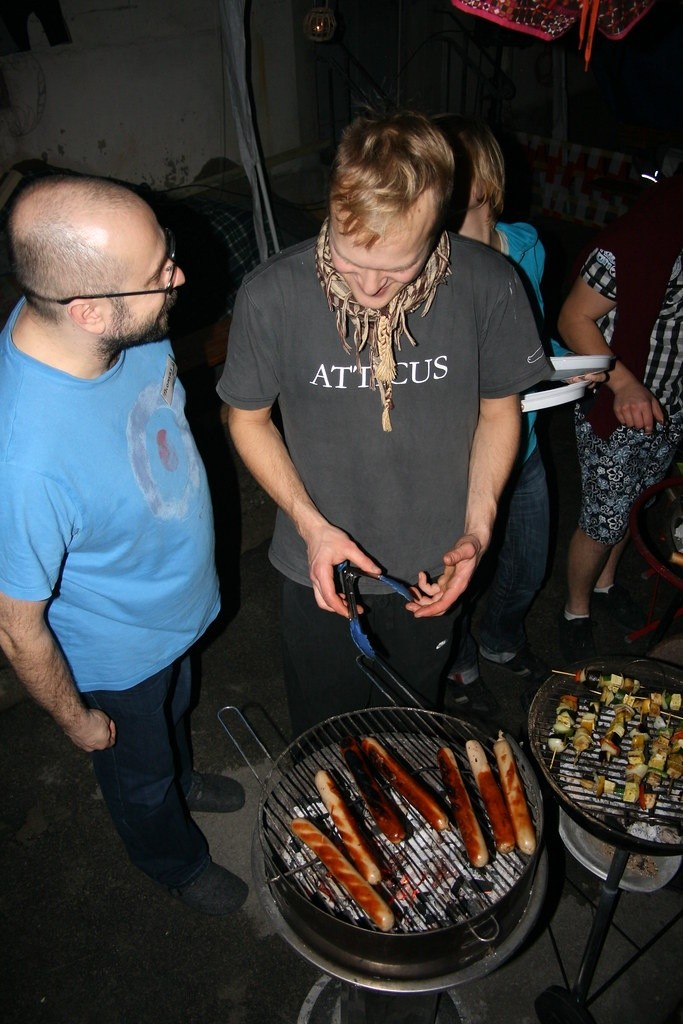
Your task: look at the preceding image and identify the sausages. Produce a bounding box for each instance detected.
[291,730,536,934]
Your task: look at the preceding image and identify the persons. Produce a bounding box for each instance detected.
[0,176,248,916]
[212,109,683,765]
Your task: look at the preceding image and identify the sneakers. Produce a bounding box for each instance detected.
[477,642,548,683]
[558,605,600,663]
[443,674,502,717]
[589,584,648,632]
[178,770,246,813]
[167,860,249,915]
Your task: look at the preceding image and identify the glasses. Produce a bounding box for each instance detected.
[62,226,178,306]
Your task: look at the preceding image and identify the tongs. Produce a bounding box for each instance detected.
[338,561,412,662]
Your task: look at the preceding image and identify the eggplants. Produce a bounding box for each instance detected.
[547,668,668,794]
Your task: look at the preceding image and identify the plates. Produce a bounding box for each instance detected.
[548,355,613,380]
[520,379,589,413]
